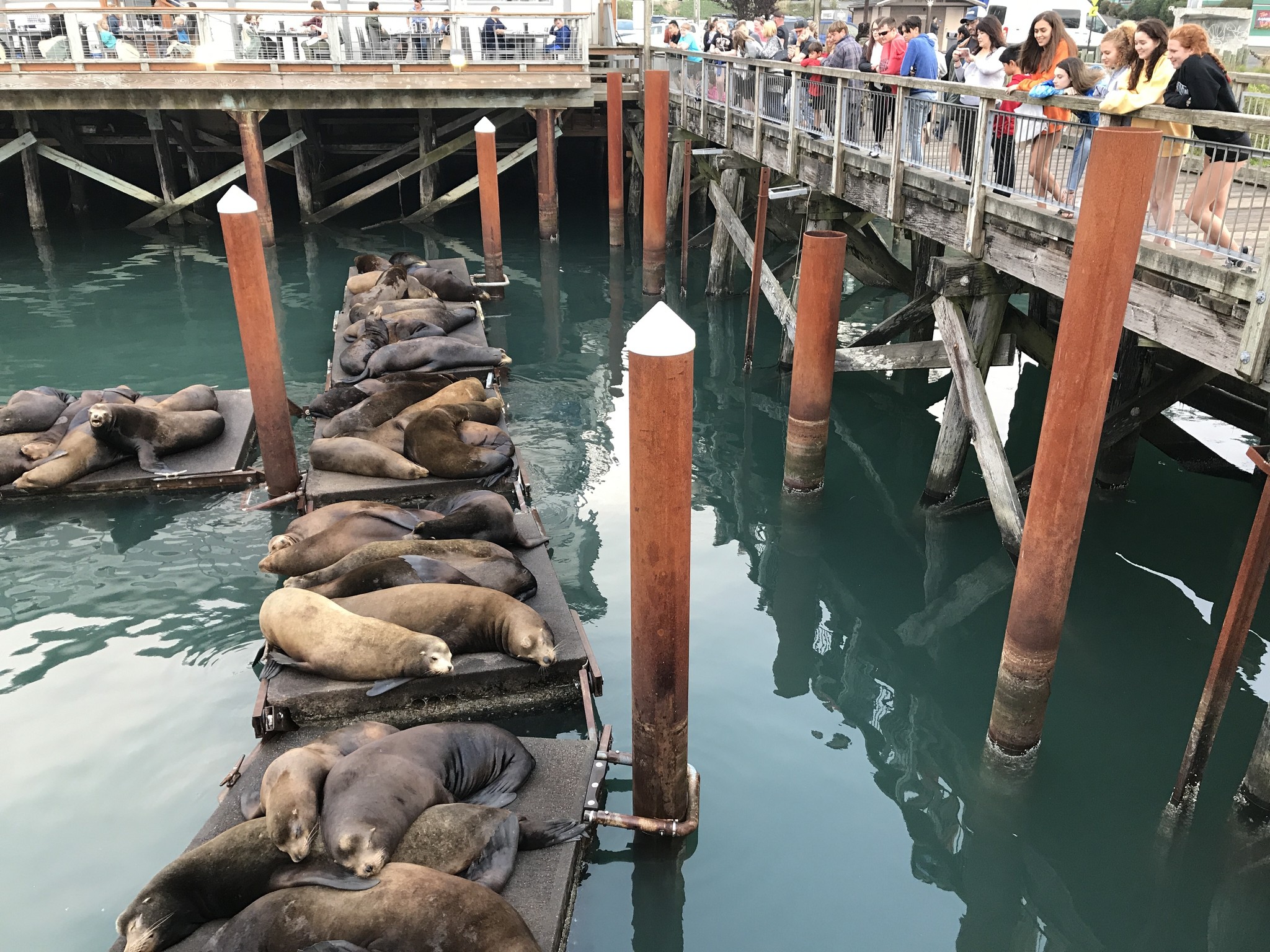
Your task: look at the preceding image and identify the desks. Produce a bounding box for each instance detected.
[260,29,317,60]
[388,31,446,60]
[0,29,51,60]
[115,26,174,58]
[502,31,553,60]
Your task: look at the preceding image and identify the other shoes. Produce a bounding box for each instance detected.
[1226,246,1253,269]
[164,54,170,58]
[1060,191,1075,219]
[807,125,823,139]
[932,127,943,141]
[873,144,883,150]
[923,128,930,144]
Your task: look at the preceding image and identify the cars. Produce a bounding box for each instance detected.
[703,13,738,32]
[616,14,705,53]
[819,20,859,47]
[783,16,804,33]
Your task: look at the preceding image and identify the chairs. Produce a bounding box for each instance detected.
[477,26,496,60]
[234,21,264,59]
[367,24,397,60]
[171,22,200,58]
[353,24,372,59]
[491,25,519,60]
[550,26,579,61]
[535,26,550,60]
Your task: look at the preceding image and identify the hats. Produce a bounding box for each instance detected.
[791,19,808,31]
[772,11,785,18]
[960,6,987,23]
[173,17,185,27]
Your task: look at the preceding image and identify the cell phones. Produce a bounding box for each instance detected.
[787,45,793,49]
[957,48,970,58]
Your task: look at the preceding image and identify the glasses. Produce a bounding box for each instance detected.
[871,28,880,31]
[878,29,891,36]
[964,19,978,28]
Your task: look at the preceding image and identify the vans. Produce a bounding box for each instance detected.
[985,0,1117,54]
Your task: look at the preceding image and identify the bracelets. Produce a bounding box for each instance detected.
[954,58,959,62]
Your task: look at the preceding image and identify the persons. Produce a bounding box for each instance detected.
[158,1,197,58]
[1099,17,1191,249]
[406,0,450,60]
[991,44,1032,198]
[481,6,515,60]
[299,1,329,59]
[543,18,571,53]
[1092,19,1139,127]
[1005,10,1078,208]
[97,4,138,49]
[664,6,1009,185]
[365,2,409,60]
[1029,57,1108,217]
[42,3,68,41]
[1163,24,1254,268]
[240,13,277,59]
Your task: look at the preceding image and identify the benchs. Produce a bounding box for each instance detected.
[93,22,118,60]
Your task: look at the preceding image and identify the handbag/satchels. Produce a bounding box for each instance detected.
[769,37,788,60]
[1013,102,1049,143]
